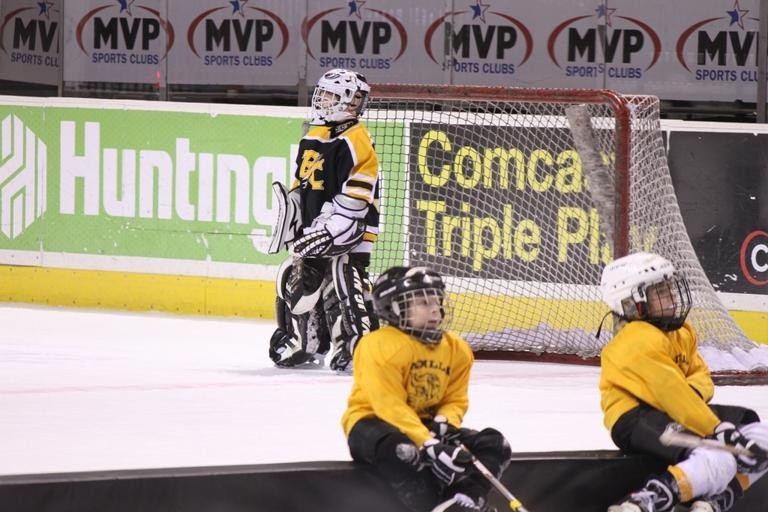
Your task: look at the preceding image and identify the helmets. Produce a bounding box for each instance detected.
[599,250,694,331]
[371,264,448,346]
[311,67,371,123]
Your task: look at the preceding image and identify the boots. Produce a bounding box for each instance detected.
[620,478,679,512]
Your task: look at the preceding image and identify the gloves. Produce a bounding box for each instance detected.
[714,418,768,476]
[399,421,475,486]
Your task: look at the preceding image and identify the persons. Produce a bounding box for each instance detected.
[596,252,768,512]
[269,70,380,375]
[339,266,512,512]
